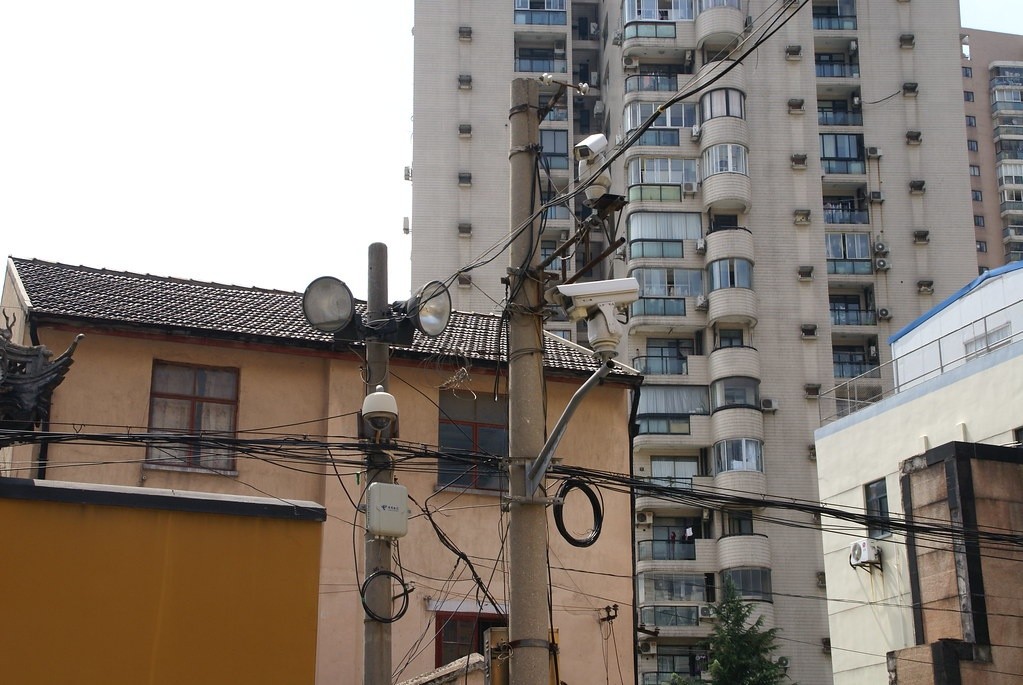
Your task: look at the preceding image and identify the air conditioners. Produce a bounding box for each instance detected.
[691,125,700,137]
[634,511,654,525]
[590,71,604,118]
[760,397,779,411]
[695,238,706,251]
[636,640,659,655]
[874,241,891,255]
[403,217,410,235]
[877,306,894,321]
[866,147,884,159]
[682,181,698,193]
[702,508,710,520]
[685,49,692,62]
[875,257,891,272]
[850,537,879,567]
[590,22,597,35]
[869,190,885,204]
[850,40,858,51]
[403,165,410,181]
[695,295,708,310]
[852,97,860,105]
[697,605,719,620]
[622,54,640,70]
[869,345,877,357]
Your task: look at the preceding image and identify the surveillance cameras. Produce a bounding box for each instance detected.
[360,392,399,430]
[543,276,639,311]
[572,133,607,161]
